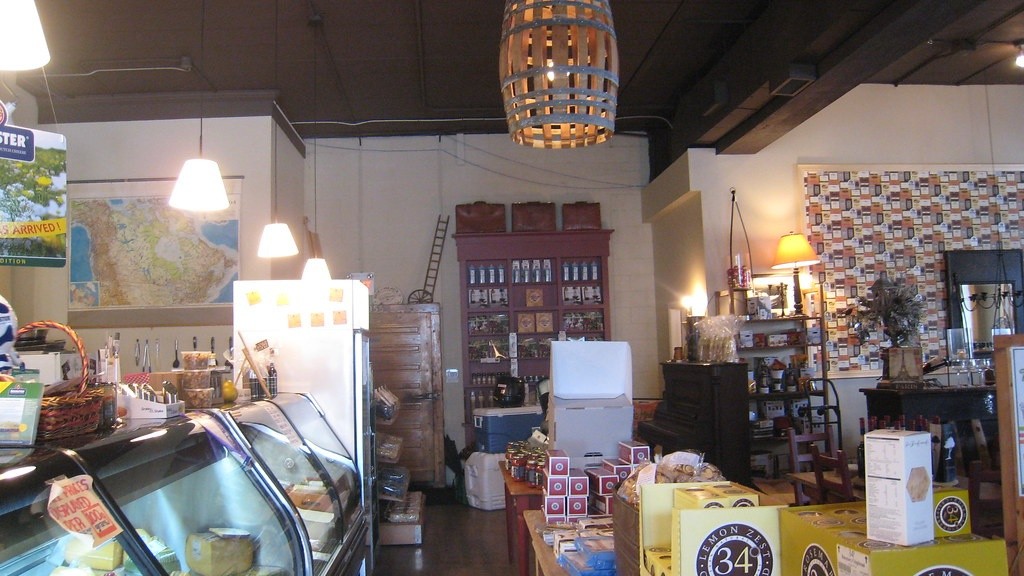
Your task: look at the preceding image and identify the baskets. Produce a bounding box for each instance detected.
[11,320,109,439]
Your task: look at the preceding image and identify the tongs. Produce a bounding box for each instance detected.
[142,339,151,373]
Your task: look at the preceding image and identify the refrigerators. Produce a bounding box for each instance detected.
[233,278,382,576]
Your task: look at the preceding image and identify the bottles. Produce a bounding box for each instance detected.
[469,261,598,284]
[923,357,949,373]
[857,414,941,479]
[268,363,277,395]
[471,372,546,406]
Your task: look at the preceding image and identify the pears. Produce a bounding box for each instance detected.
[222,378,238,402]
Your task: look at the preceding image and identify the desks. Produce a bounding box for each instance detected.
[859,385,1001,477]
[787,473,866,504]
[500,459,539,576]
[522,507,619,576]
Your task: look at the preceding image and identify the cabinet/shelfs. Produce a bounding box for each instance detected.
[727,315,840,477]
[447,229,612,428]
[369,382,426,546]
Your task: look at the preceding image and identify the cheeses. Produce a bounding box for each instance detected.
[46,528,254,576]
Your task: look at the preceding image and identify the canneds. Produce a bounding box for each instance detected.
[505,440,546,490]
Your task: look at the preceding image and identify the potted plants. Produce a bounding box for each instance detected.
[847,273,923,384]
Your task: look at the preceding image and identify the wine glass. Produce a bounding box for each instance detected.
[962,359,978,388]
[976,358,991,387]
[951,359,966,389]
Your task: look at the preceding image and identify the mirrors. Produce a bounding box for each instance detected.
[957,282,1019,355]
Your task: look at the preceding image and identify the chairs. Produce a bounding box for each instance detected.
[787,426,861,504]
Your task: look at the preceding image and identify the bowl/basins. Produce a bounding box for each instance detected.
[182,370,211,388]
[180,351,211,370]
[182,388,215,409]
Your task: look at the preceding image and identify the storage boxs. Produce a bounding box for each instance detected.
[544,442,781,576]
[472,405,544,451]
[929,489,971,538]
[865,427,934,544]
[778,503,1012,576]
[463,453,508,511]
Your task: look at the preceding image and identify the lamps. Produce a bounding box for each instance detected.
[167,2,230,211]
[503,0,621,154]
[768,232,820,317]
[258,0,299,259]
[303,16,333,290]
